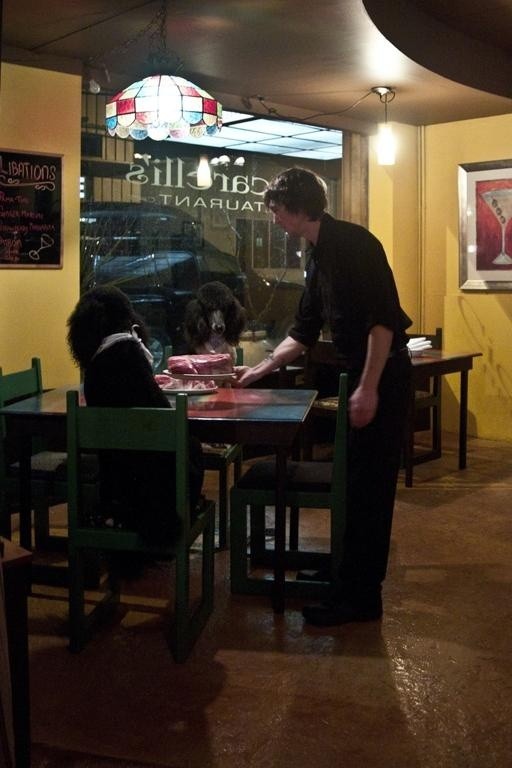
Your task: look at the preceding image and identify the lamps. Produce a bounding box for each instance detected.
[90,79,101,96]
[104,75,223,140]
[372,87,399,166]
[197,154,212,187]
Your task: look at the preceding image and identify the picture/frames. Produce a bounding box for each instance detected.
[458,160,512,293]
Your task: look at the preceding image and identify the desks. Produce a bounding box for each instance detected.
[1,385,320,619]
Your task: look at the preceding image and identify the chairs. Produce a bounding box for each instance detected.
[0,358,116,591]
[406,328,442,465]
[227,372,350,604]
[64,389,216,658]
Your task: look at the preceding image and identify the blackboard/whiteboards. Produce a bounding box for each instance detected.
[0,148,63,270]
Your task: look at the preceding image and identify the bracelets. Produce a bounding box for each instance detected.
[268,353,281,369]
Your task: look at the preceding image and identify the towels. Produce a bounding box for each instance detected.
[408,336,433,353]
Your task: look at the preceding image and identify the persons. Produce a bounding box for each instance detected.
[230,167,416,629]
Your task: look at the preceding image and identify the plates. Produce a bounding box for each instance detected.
[160,385,221,394]
[161,368,237,381]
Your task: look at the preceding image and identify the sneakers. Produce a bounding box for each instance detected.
[295,567,383,627]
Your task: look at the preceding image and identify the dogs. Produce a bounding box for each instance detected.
[181,279,248,370]
[61,284,207,597]
